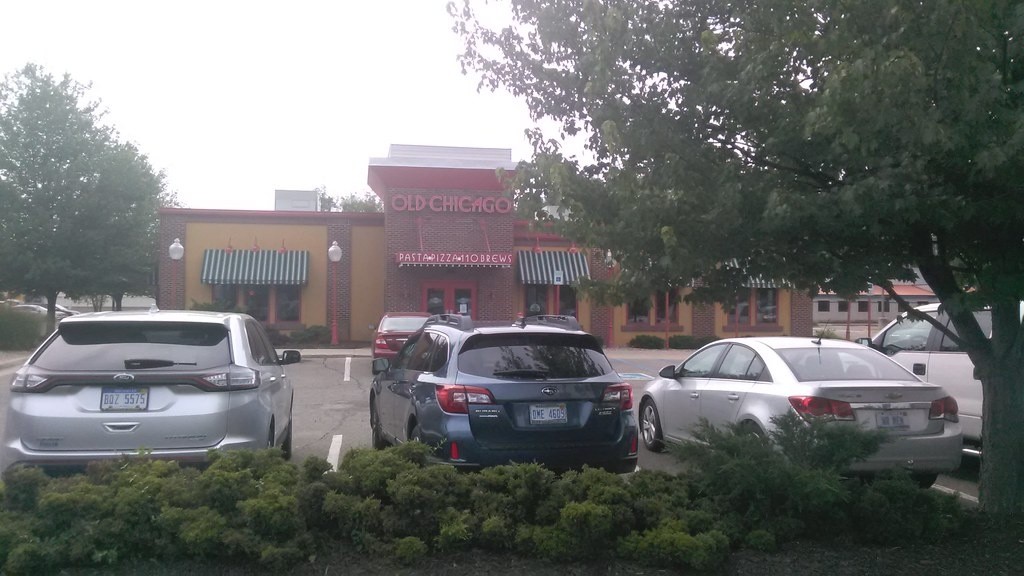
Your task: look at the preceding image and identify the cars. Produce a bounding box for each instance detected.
[36,304,80,316]
[12,305,67,320]
[839,303,1024,457]
[368,313,433,359]
[5,309,302,478]
[635,335,963,490]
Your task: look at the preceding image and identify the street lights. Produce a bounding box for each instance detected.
[328,241,342,346]
[605,249,617,348]
[169,237,184,309]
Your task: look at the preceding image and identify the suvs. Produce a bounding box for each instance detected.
[368,313,639,475]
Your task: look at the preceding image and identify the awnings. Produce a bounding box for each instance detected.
[620,256,796,288]
[200,248,309,286]
[517,250,591,285]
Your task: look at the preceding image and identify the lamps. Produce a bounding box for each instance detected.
[328,241,342,262]
[169,237,184,260]
